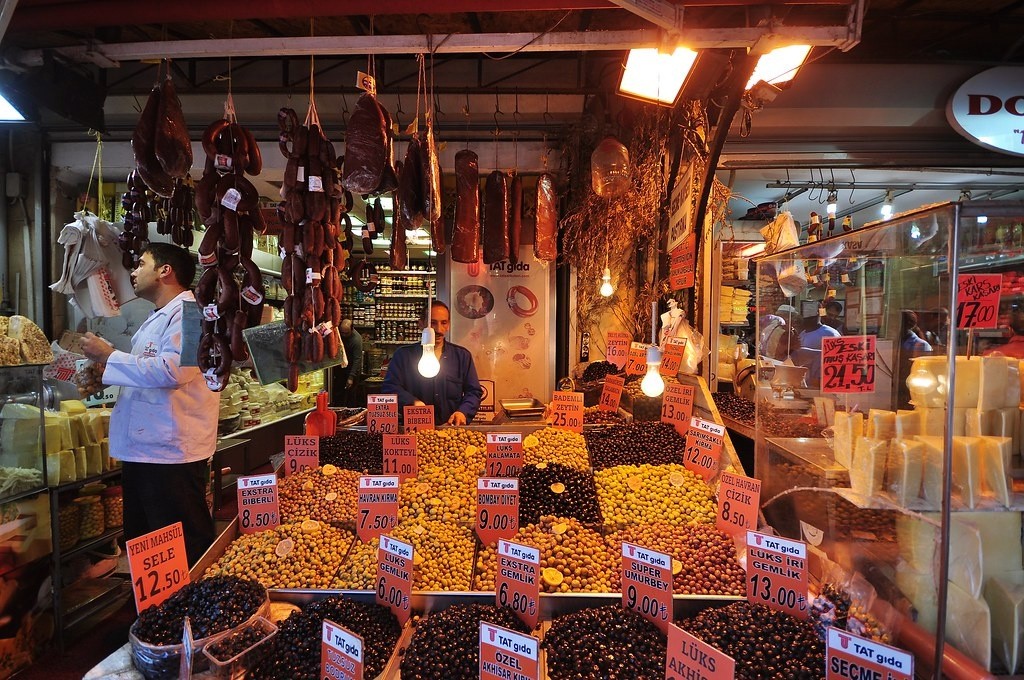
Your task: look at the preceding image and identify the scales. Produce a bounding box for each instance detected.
[492,398,547,425]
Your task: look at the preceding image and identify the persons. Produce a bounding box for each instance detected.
[78,241,221,574]
[332,319,362,408]
[900,311,933,352]
[380,300,483,426]
[982,303,1024,404]
[744,302,847,383]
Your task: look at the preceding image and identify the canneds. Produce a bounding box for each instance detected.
[341,275,436,342]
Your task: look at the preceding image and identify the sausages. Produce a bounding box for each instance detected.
[118,105,383,393]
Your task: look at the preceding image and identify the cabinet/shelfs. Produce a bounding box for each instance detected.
[720,280,751,326]
[0,359,134,680]
[942,261,1024,332]
[214,438,251,510]
[340,271,437,344]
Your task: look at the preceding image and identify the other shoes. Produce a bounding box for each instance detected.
[104,616,138,644]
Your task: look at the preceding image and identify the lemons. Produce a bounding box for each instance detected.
[411,432,478,565]
[627,473,684,575]
[524,435,566,586]
[276,464,337,556]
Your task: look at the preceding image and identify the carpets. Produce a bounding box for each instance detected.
[214,462,275,519]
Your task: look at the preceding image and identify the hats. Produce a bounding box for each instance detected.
[801,302,818,318]
[778,304,798,314]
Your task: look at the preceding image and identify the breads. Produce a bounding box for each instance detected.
[0,315,55,366]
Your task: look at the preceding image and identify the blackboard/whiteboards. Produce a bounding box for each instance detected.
[242,320,348,387]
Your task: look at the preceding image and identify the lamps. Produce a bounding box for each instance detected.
[742,45,814,91]
[881,188,894,215]
[0,69,39,124]
[47,62,112,136]
[614,46,703,109]
[827,196,836,213]
